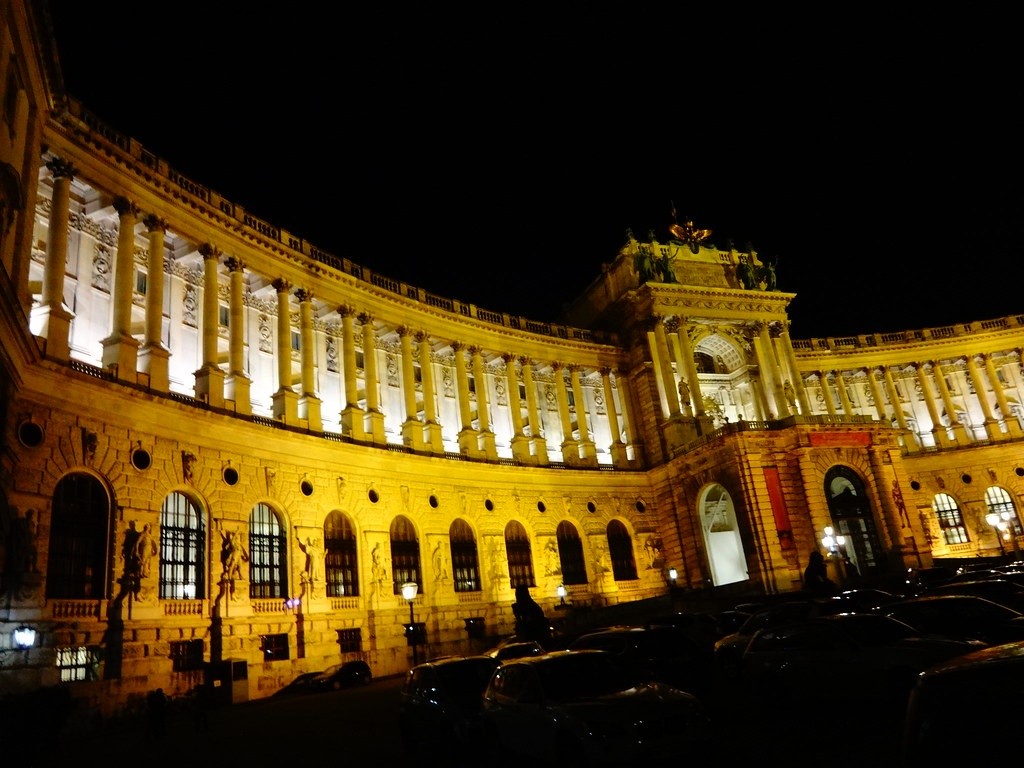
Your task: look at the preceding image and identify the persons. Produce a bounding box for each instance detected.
[784,378,796,406]
[373,543,387,580]
[678,377,691,407]
[634,242,656,286]
[432,543,450,581]
[595,541,610,573]
[13,508,44,575]
[644,539,657,569]
[803,552,827,602]
[512,584,545,642]
[545,543,558,575]
[758,256,777,289]
[305,537,328,582]
[656,246,679,282]
[736,253,758,289]
[491,545,509,579]
[226,525,249,580]
[136,523,158,578]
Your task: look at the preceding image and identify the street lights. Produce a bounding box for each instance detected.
[401,582,420,667]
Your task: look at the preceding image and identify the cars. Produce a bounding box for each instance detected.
[309,659,373,692]
[402,558,1023,767]
